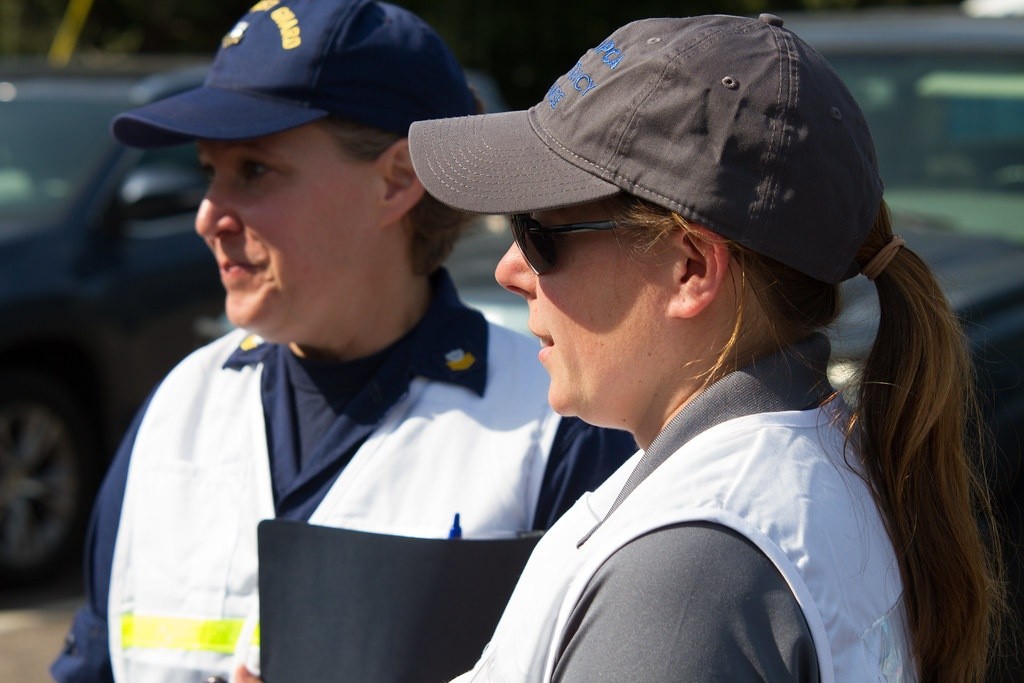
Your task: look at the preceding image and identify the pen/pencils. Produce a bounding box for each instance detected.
[447,512,465,539]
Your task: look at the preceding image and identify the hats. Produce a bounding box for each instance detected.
[407,13,882,284]
[111,0,479,148]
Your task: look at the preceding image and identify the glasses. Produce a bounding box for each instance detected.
[509,213,648,275]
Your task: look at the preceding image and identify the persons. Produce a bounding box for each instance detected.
[52,1,635,683]
[405,13,994,683]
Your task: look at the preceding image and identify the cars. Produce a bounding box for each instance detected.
[440,8,1024,547]
[0,53,234,606]
